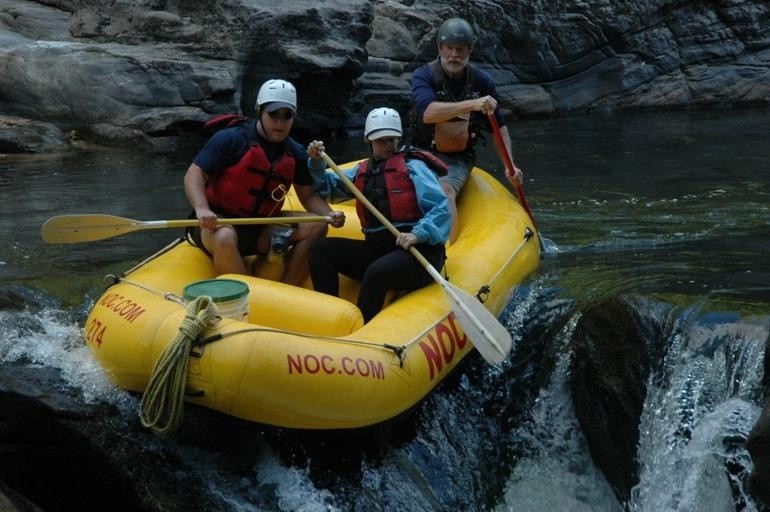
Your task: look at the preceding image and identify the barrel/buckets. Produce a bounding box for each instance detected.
[184,279,250,323]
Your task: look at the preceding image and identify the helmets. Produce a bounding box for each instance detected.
[437,18,474,45]
[364,107,403,143]
[255,79,298,109]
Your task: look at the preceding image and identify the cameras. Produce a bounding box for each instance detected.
[270,222,299,254]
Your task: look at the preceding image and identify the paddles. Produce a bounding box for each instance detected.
[39,212,343,245]
[478,96,559,255]
[312,140,514,364]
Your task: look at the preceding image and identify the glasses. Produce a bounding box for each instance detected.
[268,110,293,119]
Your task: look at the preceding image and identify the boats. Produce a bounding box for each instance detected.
[83,148,542,431]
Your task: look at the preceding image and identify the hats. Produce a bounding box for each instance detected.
[368,129,402,141]
[260,102,297,115]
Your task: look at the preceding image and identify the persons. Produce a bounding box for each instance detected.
[307,104,454,326]
[181,79,348,287]
[396,15,525,249]
